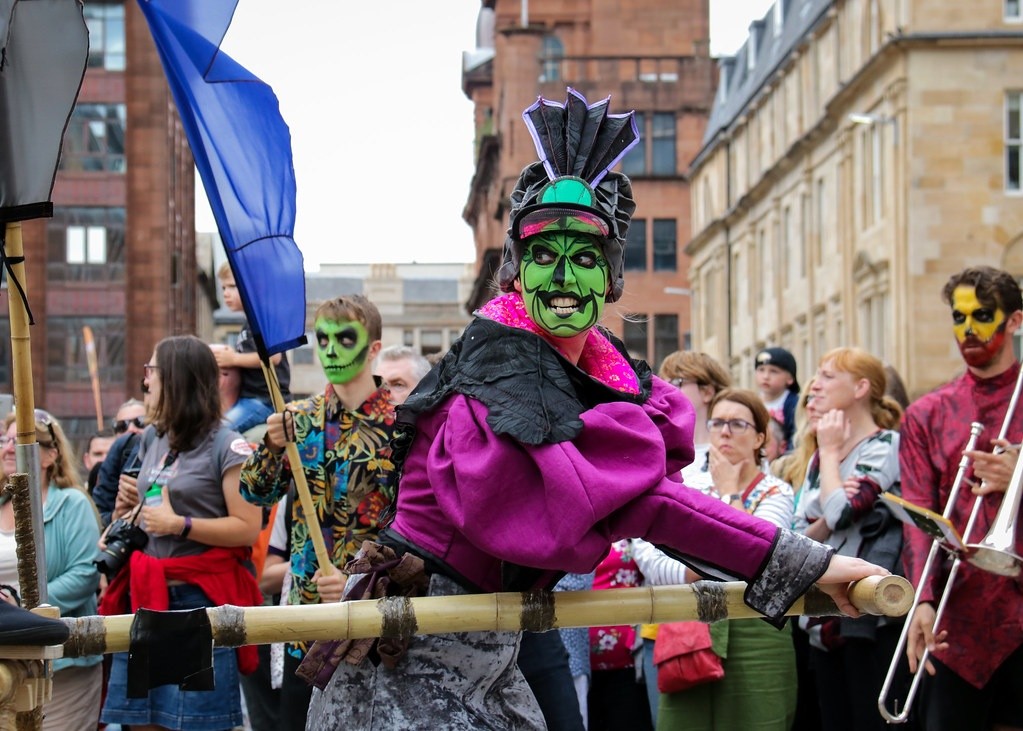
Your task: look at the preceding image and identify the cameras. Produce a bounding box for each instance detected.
[92,519,149,578]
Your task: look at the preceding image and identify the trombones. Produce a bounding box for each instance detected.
[877,361,1023,725]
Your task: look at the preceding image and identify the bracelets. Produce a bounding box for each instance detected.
[180,516,192,538]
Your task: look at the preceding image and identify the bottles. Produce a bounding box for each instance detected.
[144,483,170,538]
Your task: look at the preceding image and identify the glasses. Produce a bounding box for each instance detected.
[706,416,758,433]
[144,364,160,378]
[11,405,57,448]
[0,435,18,448]
[112,416,147,434]
[668,379,701,389]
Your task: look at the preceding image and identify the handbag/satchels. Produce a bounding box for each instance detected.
[652,613,725,693]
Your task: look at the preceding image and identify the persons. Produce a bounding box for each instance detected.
[0,408,104,731]
[549,349,912,731]
[83,263,290,731]
[237,295,400,731]
[372,347,431,406]
[294,86,894,730]
[896,265,1023,731]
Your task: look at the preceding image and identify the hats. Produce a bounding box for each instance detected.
[756,348,800,392]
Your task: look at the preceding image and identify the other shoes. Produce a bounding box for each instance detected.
[0,585,70,646]
